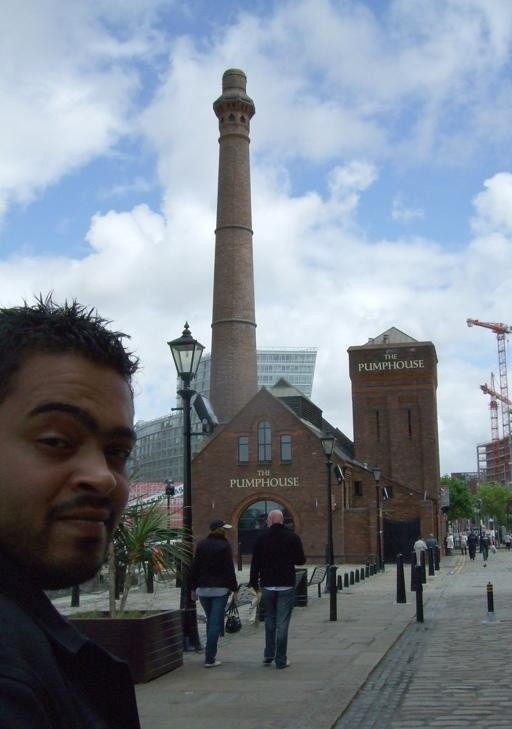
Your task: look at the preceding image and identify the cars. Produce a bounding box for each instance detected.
[154,538,183,545]
[452,527,497,547]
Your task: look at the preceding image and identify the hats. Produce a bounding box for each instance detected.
[209,520,232,529]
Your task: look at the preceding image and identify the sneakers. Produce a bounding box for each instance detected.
[263,657,290,670]
[204,661,222,667]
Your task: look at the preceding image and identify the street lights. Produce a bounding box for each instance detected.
[371,465,385,571]
[477,497,483,539]
[165,319,221,656]
[319,428,338,595]
[163,477,175,545]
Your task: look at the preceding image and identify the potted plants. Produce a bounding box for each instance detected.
[65,493,195,684]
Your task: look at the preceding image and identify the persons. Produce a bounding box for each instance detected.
[0,292,139,729]
[445,531,512,561]
[414,533,437,566]
[190,521,239,668]
[250,509,307,669]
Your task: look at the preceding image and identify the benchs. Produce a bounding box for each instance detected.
[307,564,329,598]
[224,583,256,616]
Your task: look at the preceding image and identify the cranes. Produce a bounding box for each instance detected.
[462,314,512,489]
[475,368,512,481]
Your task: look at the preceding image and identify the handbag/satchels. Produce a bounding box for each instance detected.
[226,616,242,633]
